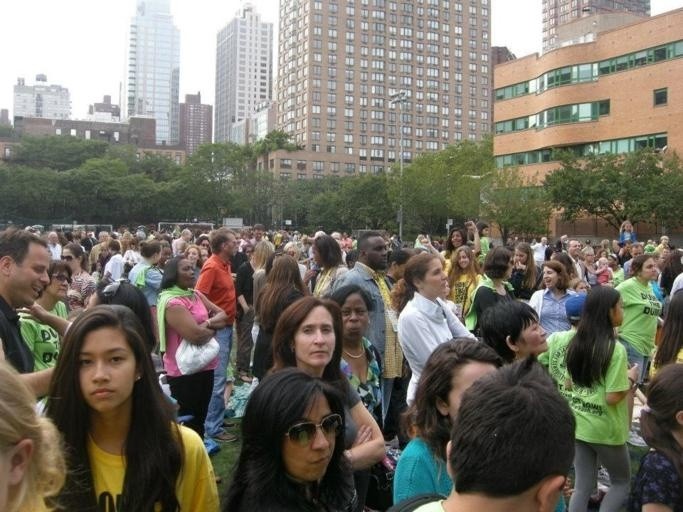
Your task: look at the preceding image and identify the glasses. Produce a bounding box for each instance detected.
[285,414,343,445]
[103,278,129,302]
[61,256,74,262]
[51,273,72,284]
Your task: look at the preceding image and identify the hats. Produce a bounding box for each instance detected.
[566,294,585,320]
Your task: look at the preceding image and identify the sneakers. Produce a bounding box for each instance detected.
[215,433,237,442]
[223,419,234,426]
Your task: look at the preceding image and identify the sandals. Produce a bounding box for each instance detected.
[238,373,253,383]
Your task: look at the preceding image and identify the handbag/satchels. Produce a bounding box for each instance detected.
[176,336,220,376]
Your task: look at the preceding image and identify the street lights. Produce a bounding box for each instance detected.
[389,90,408,241]
